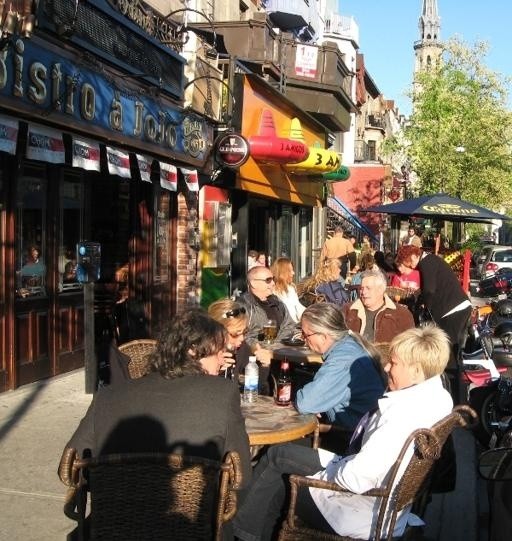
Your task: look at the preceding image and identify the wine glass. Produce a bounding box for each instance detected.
[394,289,402,304]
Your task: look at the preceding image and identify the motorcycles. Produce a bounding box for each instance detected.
[464,280,512,488]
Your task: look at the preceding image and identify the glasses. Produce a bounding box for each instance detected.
[253,276,275,284]
[223,305,247,322]
[300,330,322,342]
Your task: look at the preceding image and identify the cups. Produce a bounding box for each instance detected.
[263,317,277,346]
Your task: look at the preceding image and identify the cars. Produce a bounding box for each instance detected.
[469,239,512,280]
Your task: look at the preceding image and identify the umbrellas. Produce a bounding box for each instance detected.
[357,190,512,258]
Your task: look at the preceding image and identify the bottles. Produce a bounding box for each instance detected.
[244,354,259,407]
[275,354,292,407]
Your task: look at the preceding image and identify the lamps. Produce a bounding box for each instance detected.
[154,5,219,60]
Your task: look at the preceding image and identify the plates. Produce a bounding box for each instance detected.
[281,339,305,346]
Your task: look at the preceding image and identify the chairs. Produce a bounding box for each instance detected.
[57,446,242,541]
[277,404,478,539]
[117,340,158,380]
[387,287,422,323]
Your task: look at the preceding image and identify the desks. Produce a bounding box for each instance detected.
[255,342,326,379]
[238,394,320,467]
